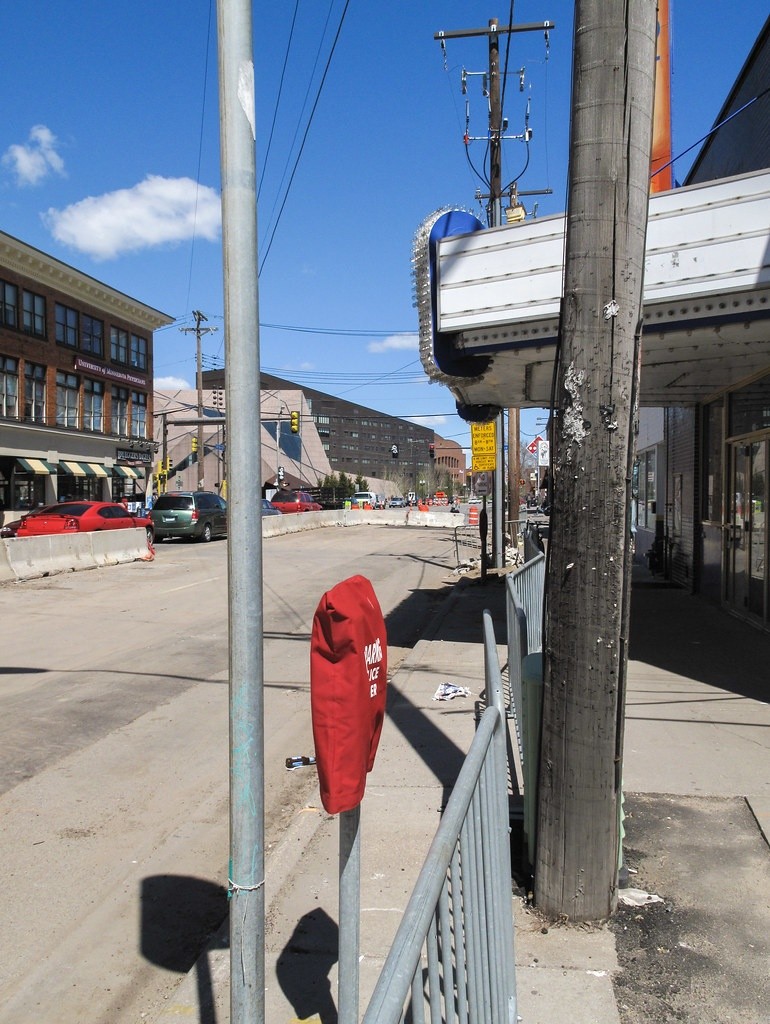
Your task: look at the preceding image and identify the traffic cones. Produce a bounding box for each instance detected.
[469,506,478,525]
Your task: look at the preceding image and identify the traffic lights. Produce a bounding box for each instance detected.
[430,444,434,458]
[166,456,173,471]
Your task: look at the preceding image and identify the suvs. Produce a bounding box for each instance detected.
[147,490,228,543]
[389,496,406,508]
[270,491,323,514]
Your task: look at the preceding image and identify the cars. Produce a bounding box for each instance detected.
[261,499,283,516]
[468,497,482,504]
[0,501,154,549]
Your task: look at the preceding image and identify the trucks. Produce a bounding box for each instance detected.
[354,492,386,510]
